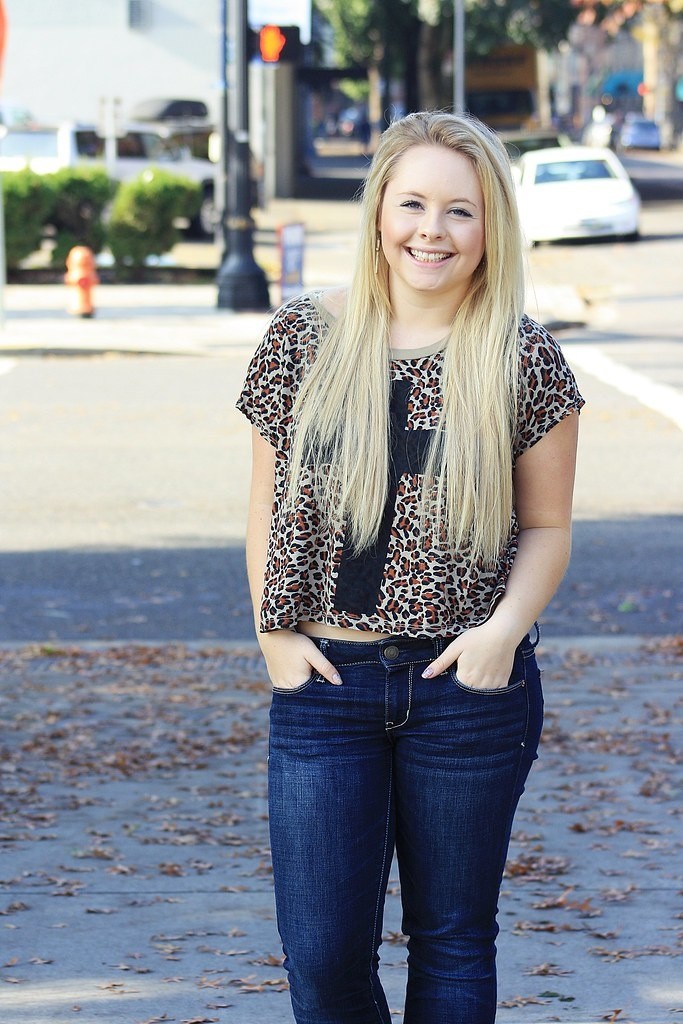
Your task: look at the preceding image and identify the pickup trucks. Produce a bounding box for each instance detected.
[0,125,213,202]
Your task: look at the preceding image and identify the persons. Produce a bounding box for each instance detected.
[359,116,372,159]
[238,108,581,1024]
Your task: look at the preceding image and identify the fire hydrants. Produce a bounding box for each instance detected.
[63,246,98,318]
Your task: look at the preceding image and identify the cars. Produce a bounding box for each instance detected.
[515,145,640,247]
[621,115,659,150]
[125,96,207,120]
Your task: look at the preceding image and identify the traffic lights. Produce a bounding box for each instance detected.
[257,26,303,65]
